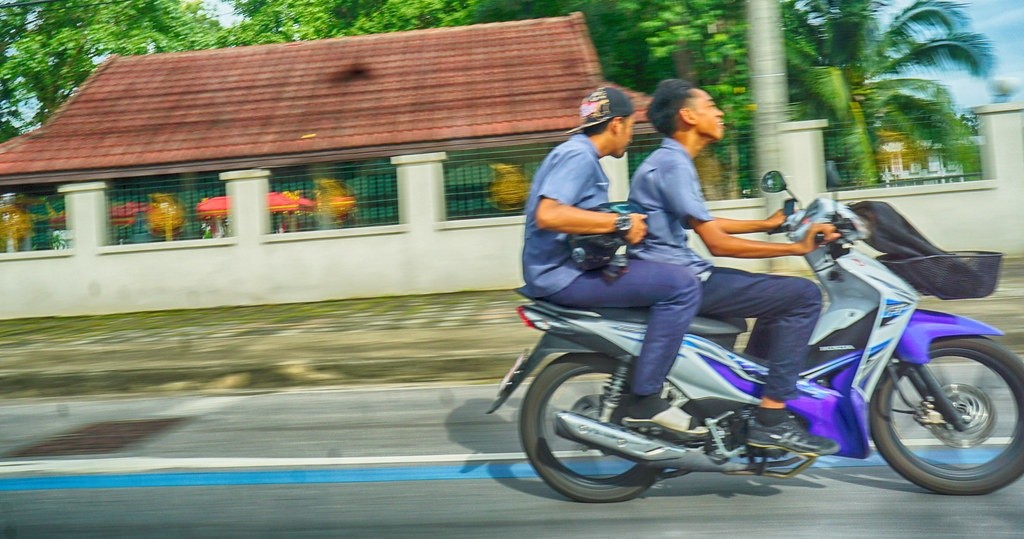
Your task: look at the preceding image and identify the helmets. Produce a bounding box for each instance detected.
[567,201,645,270]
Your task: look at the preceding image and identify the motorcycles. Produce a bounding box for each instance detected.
[483,169,1024,504]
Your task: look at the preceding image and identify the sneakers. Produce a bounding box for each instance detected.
[747,420,838,454]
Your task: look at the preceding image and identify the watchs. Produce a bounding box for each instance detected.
[615,213,632,234]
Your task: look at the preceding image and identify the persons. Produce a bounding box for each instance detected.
[626,79,840,457]
[522,87,709,438]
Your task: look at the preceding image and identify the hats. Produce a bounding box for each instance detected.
[565,87,633,135]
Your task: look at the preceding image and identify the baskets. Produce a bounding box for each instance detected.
[876,250,1003,299]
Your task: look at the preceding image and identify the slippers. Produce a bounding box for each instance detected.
[622,396,710,437]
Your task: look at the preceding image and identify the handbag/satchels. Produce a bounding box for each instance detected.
[848,200,982,297]
